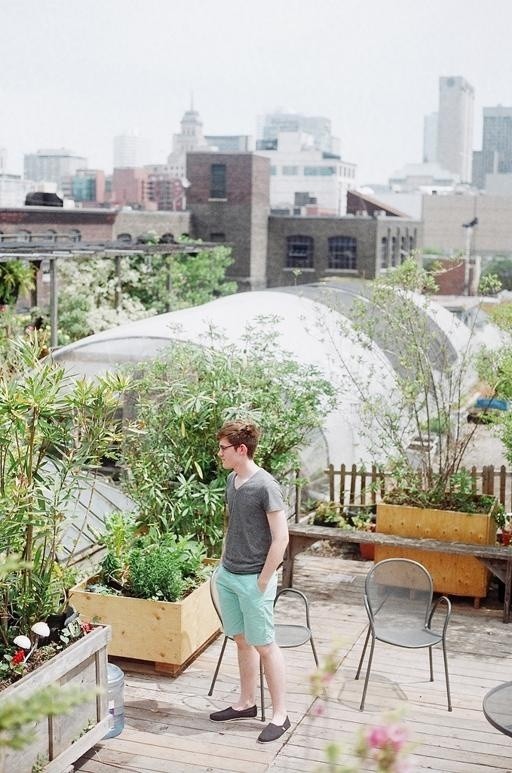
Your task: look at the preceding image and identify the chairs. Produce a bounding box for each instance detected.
[209,565,320,722]
[354,558,453,712]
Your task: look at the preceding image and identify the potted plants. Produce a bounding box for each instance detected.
[314,247,512,608]
[0,328,113,773]
[495,516,512,546]
[352,506,376,559]
[68,311,336,676]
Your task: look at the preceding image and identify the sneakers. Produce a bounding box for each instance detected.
[258,715,290,743]
[210,705,257,721]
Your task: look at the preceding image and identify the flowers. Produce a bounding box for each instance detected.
[305,653,411,771]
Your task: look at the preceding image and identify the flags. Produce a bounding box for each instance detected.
[462,217,478,228]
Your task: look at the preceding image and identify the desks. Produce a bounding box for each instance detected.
[482,680,511,737]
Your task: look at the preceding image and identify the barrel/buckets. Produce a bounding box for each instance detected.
[101,663,125,740]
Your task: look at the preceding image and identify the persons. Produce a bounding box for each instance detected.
[208,418,291,744]
